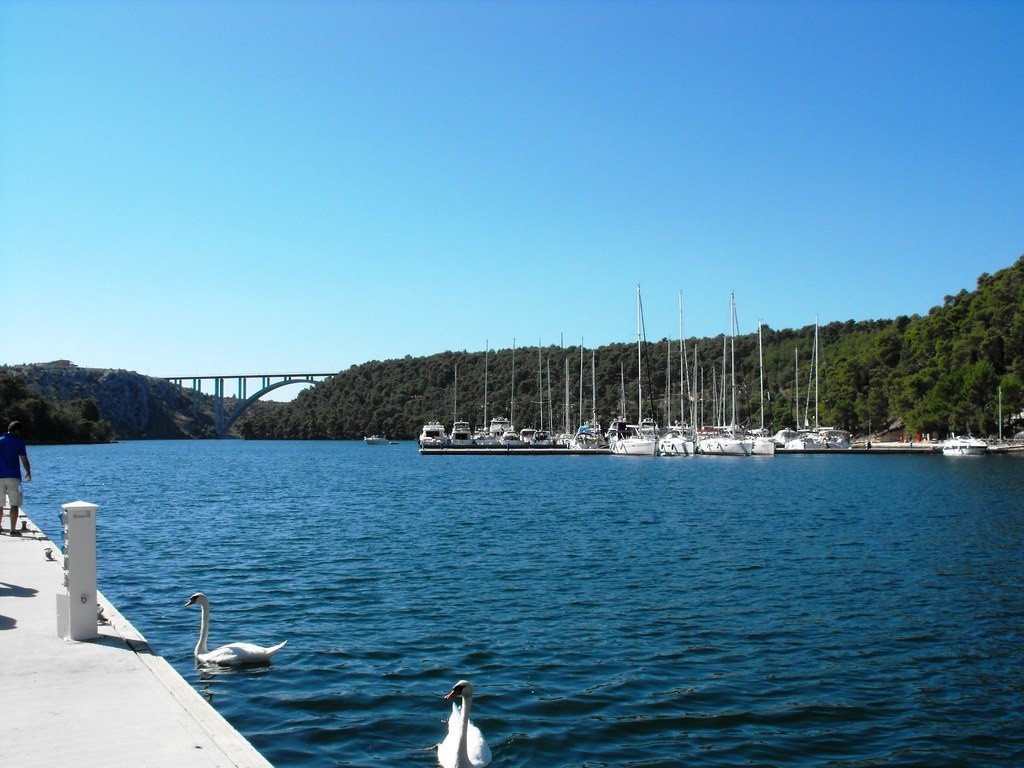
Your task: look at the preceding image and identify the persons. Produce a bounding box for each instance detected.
[0,421,31,537]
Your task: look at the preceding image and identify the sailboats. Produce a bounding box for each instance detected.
[478,280,826,457]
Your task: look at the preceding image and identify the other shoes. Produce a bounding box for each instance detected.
[0,529,6,535]
[10,529,22,537]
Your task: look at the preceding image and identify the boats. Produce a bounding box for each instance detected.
[449,419,473,447]
[418,420,449,449]
[363,434,391,445]
[389,441,401,445]
[941,435,989,457]
[1008,442,1024,453]
[988,442,1009,453]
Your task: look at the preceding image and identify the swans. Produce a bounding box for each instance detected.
[436,678,493,768]
[185,589,287,665]
